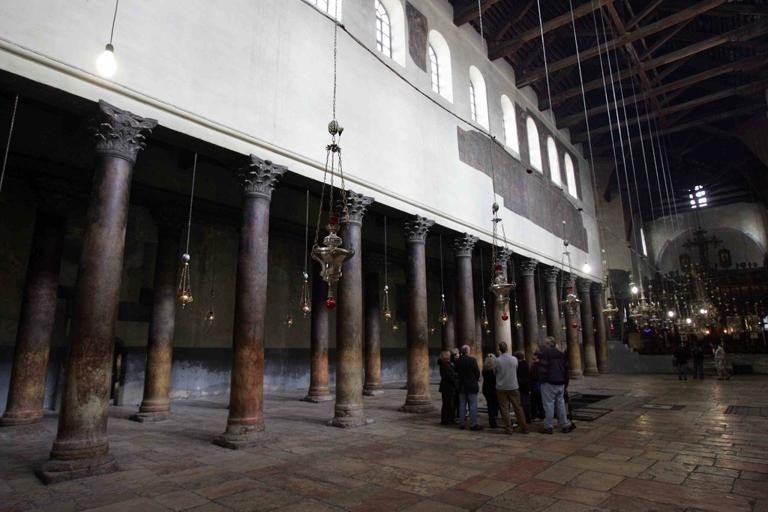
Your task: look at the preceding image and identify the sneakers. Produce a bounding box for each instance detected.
[678,374,735,381]
[442,411,576,435]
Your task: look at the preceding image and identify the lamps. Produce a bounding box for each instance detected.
[299,187,313,320]
[311,23,356,311]
[176,137,199,308]
[94,0,120,79]
[438,234,449,325]
[379,205,393,319]
[489,137,515,320]
[479,248,488,328]
[553,207,708,325]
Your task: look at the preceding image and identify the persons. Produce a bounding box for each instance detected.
[438,336,569,435]
[673,342,730,380]
